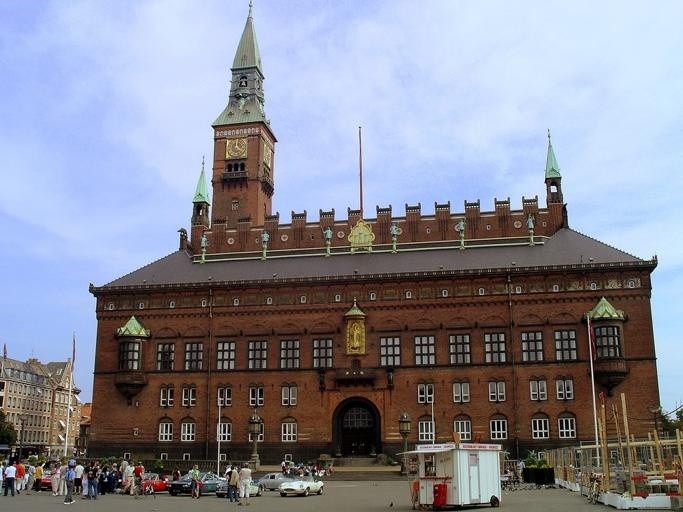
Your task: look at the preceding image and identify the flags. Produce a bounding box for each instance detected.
[588,319,596,360]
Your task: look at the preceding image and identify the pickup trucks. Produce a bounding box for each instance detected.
[257,472,293,491]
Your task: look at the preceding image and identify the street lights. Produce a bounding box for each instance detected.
[17,414,29,462]
[396,408,412,477]
[247,408,262,468]
[646,402,664,433]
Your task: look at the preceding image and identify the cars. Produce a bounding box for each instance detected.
[0,455,266,499]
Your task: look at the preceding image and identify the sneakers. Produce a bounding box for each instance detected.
[64,500,75,505]
[230,499,251,506]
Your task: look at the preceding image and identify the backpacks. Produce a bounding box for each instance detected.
[88,466,95,479]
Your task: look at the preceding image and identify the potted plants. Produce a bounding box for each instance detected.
[522,449,555,485]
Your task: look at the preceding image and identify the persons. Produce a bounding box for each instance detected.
[516,457,524,483]
[279,460,333,478]
[0,452,253,505]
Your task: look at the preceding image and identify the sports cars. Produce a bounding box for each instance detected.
[278,475,324,497]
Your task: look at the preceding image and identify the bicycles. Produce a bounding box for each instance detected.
[585,473,602,506]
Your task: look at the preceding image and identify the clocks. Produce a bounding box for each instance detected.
[225,138,247,160]
